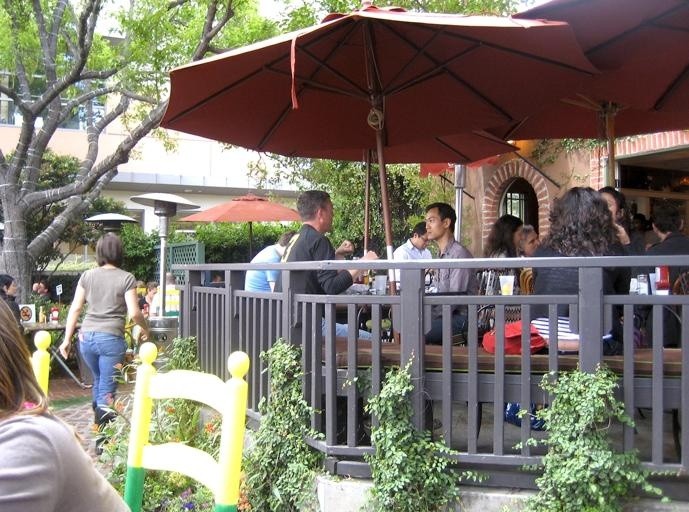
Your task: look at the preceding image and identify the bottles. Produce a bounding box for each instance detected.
[39,305,46,322]
[353,257,373,288]
[637,266,670,294]
[48,308,59,326]
[156,306,161,316]
[144,303,149,315]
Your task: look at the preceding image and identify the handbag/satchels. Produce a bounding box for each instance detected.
[483,321,546,354]
[504,402,545,431]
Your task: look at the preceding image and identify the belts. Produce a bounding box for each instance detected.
[544,346,579,355]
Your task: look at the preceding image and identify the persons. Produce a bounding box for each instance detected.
[0,274,24,336]
[59,232,149,432]
[0,296,131,512]
[131,272,180,341]
[28,279,52,322]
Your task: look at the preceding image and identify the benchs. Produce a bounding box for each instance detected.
[320,332,687,474]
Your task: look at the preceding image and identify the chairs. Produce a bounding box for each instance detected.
[23,284,183,397]
[127,341,250,512]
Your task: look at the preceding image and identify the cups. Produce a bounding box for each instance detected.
[375,276,387,293]
[499,276,514,295]
